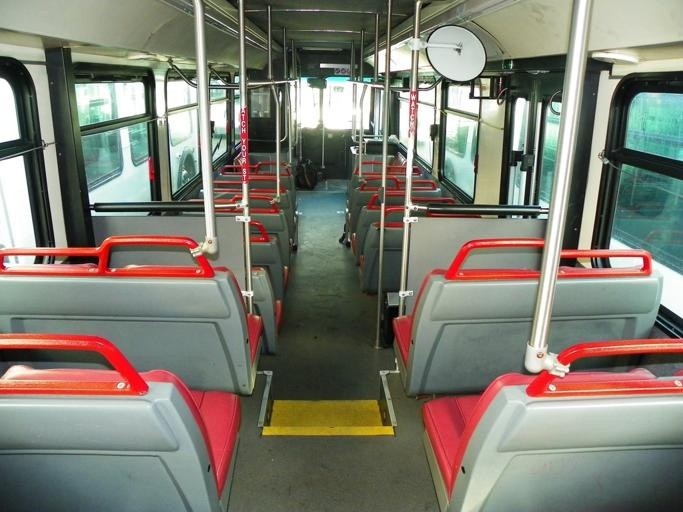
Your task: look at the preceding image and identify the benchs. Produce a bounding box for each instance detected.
[1,331,244,509]
[181,154,296,359]
[339,154,484,351]
[0,234,262,397]
[392,238,664,402]
[419,337,681,511]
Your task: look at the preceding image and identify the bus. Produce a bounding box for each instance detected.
[74,84,241,216]
[428,83,480,199]
